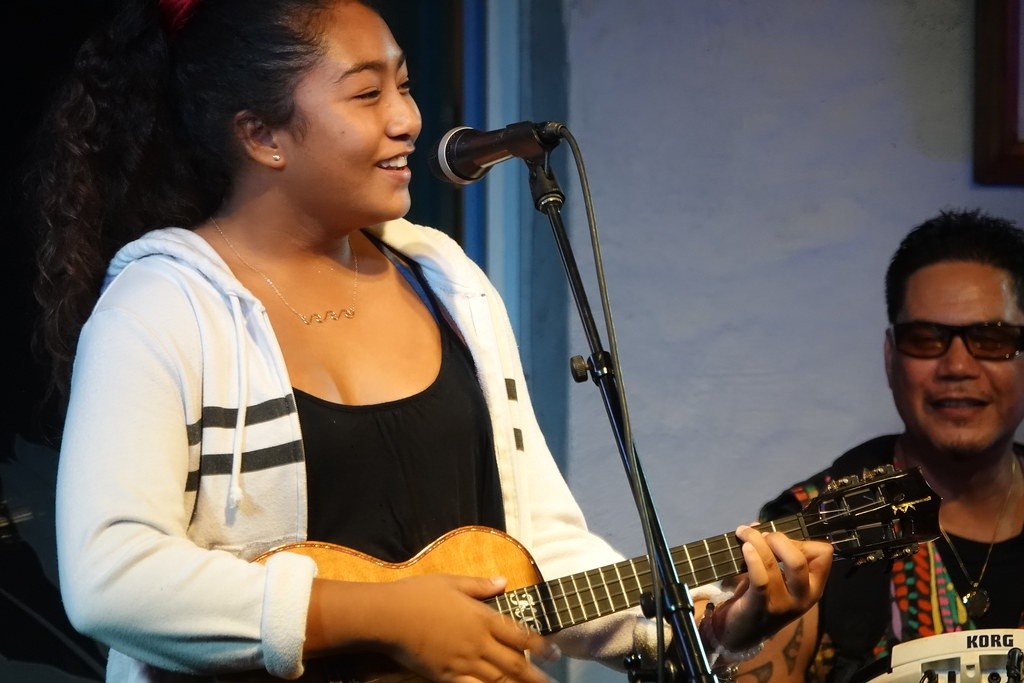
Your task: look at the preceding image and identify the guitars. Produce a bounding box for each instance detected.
[249,465,944,682]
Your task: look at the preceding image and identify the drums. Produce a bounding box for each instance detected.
[851,628,1024,683]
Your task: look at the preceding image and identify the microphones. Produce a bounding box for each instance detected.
[426,119,562,186]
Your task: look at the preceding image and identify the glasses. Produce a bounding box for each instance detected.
[884,311,1024,362]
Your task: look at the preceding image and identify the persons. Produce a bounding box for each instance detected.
[35,2,834,682]
[717,204,1024,682]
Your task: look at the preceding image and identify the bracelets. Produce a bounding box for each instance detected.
[704,603,765,660]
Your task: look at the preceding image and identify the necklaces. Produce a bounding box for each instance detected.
[907,449,1018,616]
[208,214,358,324]
